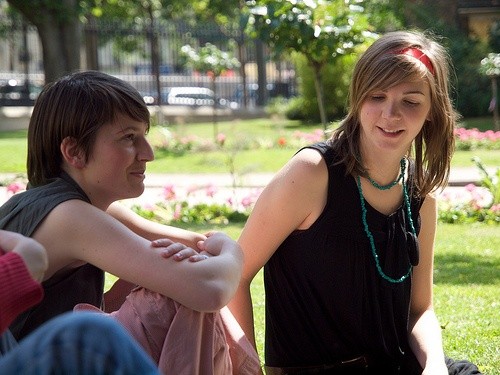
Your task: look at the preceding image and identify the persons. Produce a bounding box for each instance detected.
[227,32,451,375]
[0,71,264,375]
[0,230,163,375]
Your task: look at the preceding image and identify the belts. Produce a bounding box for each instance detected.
[285,354,369,374]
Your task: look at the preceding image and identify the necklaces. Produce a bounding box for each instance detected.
[350,159,417,283]
